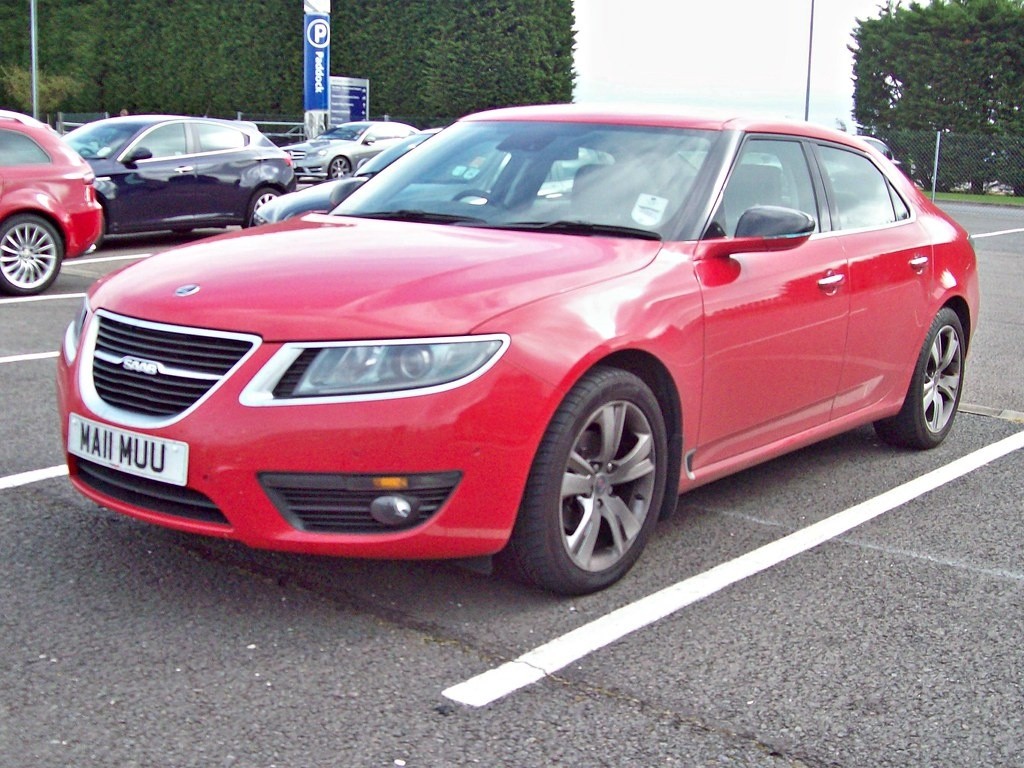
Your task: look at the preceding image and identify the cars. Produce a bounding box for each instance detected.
[279,119,421,181]
[58,100,982,598]
[0,109,105,296]
[61,115,298,235]
[253,125,613,224]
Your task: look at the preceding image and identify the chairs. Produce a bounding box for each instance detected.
[564,162,627,220]
[721,162,784,238]
